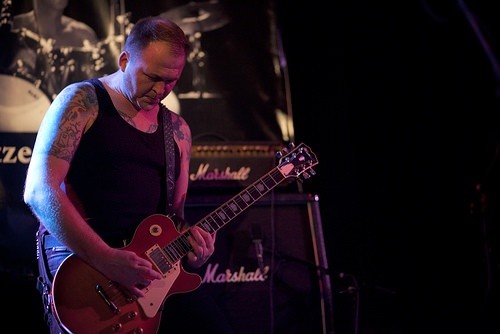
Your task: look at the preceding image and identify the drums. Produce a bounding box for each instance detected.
[0,73,54,184]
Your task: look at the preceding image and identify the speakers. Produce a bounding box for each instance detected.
[157,194,334,334]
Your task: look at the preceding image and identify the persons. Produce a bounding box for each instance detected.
[24,18,216,334]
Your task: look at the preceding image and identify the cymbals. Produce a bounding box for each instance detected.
[151,1,231,39]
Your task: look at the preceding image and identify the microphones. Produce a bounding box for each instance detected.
[248,222,266,275]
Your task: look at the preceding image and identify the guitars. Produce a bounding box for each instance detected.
[48,141,320,334]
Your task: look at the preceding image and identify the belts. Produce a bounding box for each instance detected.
[43,234,131,249]
